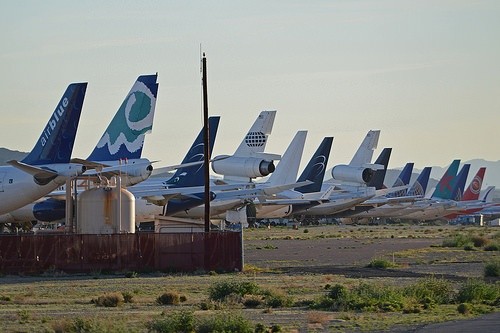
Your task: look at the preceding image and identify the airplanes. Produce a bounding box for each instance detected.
[0,72,500,230]
